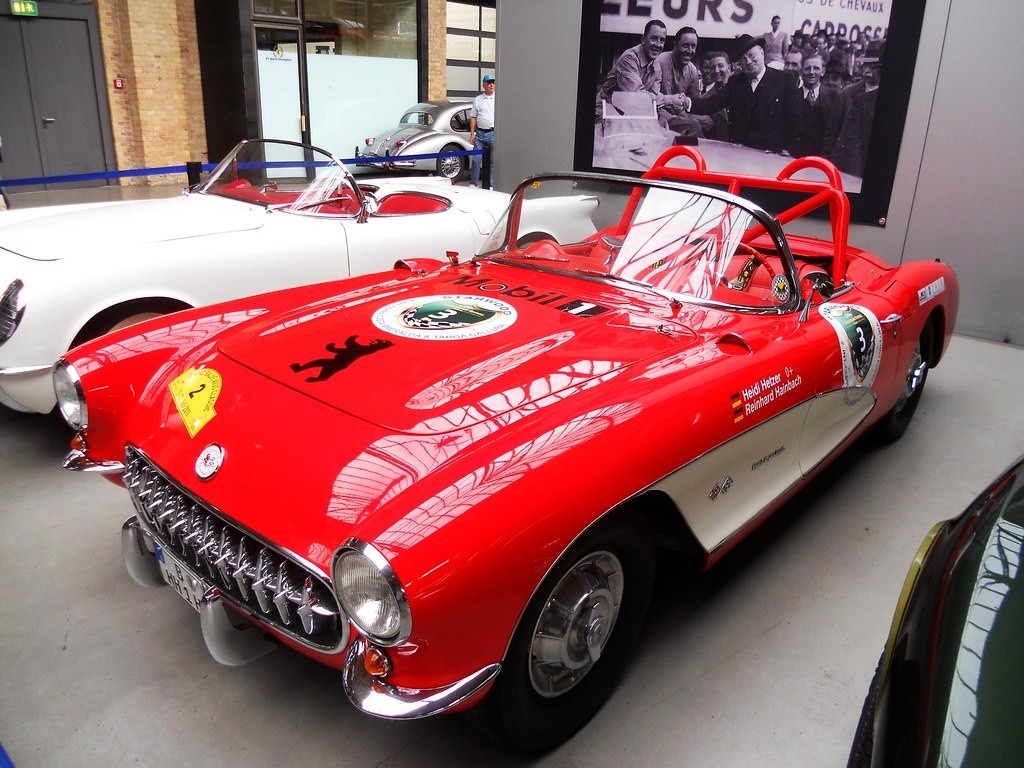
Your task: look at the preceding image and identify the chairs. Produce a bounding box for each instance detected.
[588,235,828,304]
[318,184,380,214]
[375,190,453,214]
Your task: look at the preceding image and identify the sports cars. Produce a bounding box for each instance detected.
[0,136,593,417]
[355,96,481,187]
[845,455,1024,768]
[51,142,958,761]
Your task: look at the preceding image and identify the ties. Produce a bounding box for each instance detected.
[750,78,758,92]
[806,90,816,106]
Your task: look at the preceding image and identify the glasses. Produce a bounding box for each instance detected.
[737,49,759,64]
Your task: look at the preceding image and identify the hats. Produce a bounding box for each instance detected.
[482,75,495,83]
[732,34,766,61]
[854,39,885,62]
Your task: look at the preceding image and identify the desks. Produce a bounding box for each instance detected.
[592,134,862,194]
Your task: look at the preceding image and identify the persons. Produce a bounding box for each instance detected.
[595,15,886,177]
[467,75,495,190]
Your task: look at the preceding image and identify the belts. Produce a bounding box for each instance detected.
[478,127,494,133]
